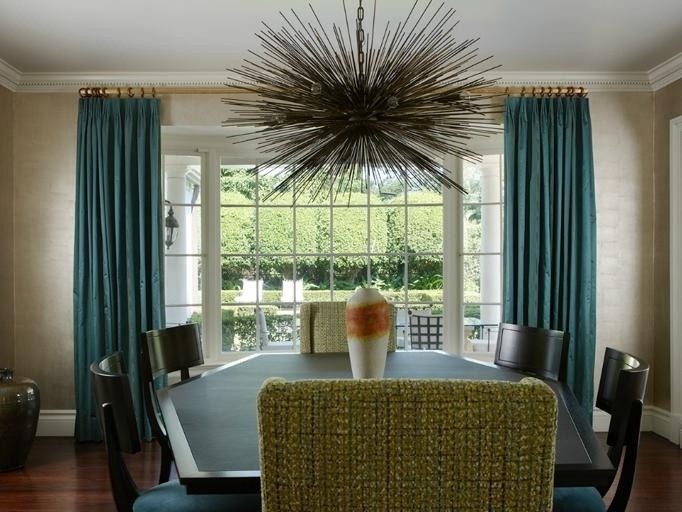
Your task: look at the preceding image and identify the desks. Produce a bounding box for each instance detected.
[156,349,617,498]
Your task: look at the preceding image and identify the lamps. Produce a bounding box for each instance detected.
[221,0,509,209]
[165,200,179,250]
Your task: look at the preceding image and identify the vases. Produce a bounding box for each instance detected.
[345,288,390,378]
[0,368,41,473]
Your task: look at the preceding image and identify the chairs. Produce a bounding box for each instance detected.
[256,378,558,512]
[554,347,650,512]
[139,323,204,484]
[298,302,397,354]
[256,308,299,351]
[494,322,570,382]
[90,351,261,512]
